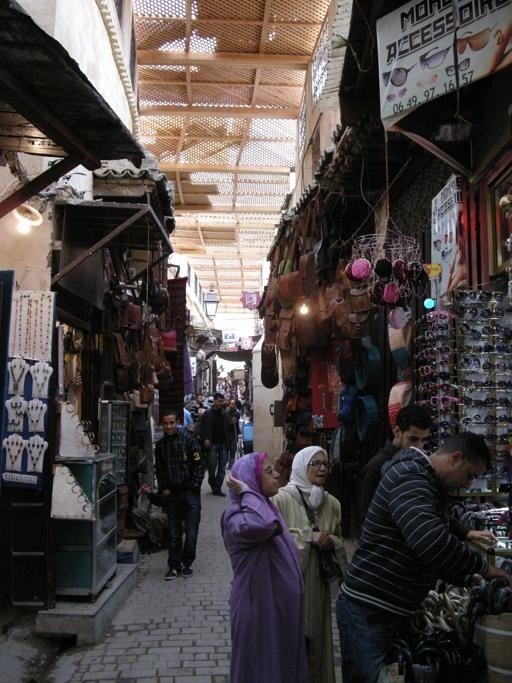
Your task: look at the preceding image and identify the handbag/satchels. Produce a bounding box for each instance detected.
[349,287,369,308]
[383,283,400,306]
[296,487,342,580]
[263,197,324,319]
[370,280,385,306]
[348,314,370,339]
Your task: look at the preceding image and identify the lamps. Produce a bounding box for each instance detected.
[13,197,45,233]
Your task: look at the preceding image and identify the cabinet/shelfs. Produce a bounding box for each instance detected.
[406,283,512,508]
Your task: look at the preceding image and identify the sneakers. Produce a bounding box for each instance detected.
[182,566,193,577]
[164,568,180,580]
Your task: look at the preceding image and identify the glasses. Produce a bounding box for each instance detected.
[459,357,479,370]
[461,378,481,388]
[462,345,482,354]
[459,412,482,426]
[483,327,505,337]
[481,309,504,317]
[485,344,506,354]
[455,306,477,317]
[308,460,328,469]
[459,396,481,409]
[480,293,503,301]
[505,380,512,389]
[506,328,512,338]
[505,344,512,353]
[485,380,506,390]
[459,324,482,339]
[485,415,507,428]
[508,364,512,371]
[485,397,508,409]
[490,448,505,457]
[482,362,507,375]
[486,433,506,443]
[455,292,476,301]
[508,416,512,425]
[412,310,455,451]
[462,457,478,482]
[488,466,507,473]
[476,433,484,440]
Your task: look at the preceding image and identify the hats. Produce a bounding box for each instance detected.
[393,259,407,279]
[351,258,370,278]
[374,259,391,275]
[345,263,361,282]
[389,308,408,328]
[407,260,427,284]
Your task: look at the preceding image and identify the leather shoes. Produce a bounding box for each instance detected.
[208,480,216,489]
[212,488,225,496]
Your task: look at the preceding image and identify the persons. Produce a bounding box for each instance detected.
[271,445,343,682]
[356,404,433,536]
[220,451,310,683]
[183,384,214,425]
[200,393,232,497]
[155,412,206,580]
[224,393,252,433]
[336,431,492,683]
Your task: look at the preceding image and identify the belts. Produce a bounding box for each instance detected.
[339,592,366,608]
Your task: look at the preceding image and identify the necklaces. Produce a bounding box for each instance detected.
[9,298,19,360]
[7,441,23,465]
[28,407,43,427]
[19,295,28,360]
[33,369,49,394]
[29,299,37,366]
[10,366,25,391]
[40,293,52,354]
[27,443,44,469]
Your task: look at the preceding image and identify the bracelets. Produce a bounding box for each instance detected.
[77,495,86,503]
[71,413,80,423]
[61,465,69,475]
[66,402,75,413]
[66,474,76,484]
[72,484,81,493]
[75,420,100,455]
[83,504,91,513]
